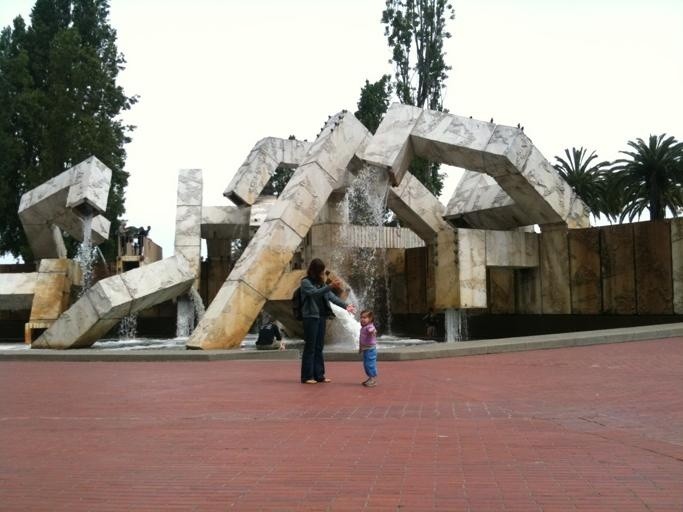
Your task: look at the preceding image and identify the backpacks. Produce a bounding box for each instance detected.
[292,276,313,321]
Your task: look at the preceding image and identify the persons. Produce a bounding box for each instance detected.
[255,315,282,350]
[422,307,436,338]
[358,309,379,387]
[138,225,151,255]
[300,257,356,384]
[118,222,131,255]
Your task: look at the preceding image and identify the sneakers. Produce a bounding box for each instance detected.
[363,377,377,387]
